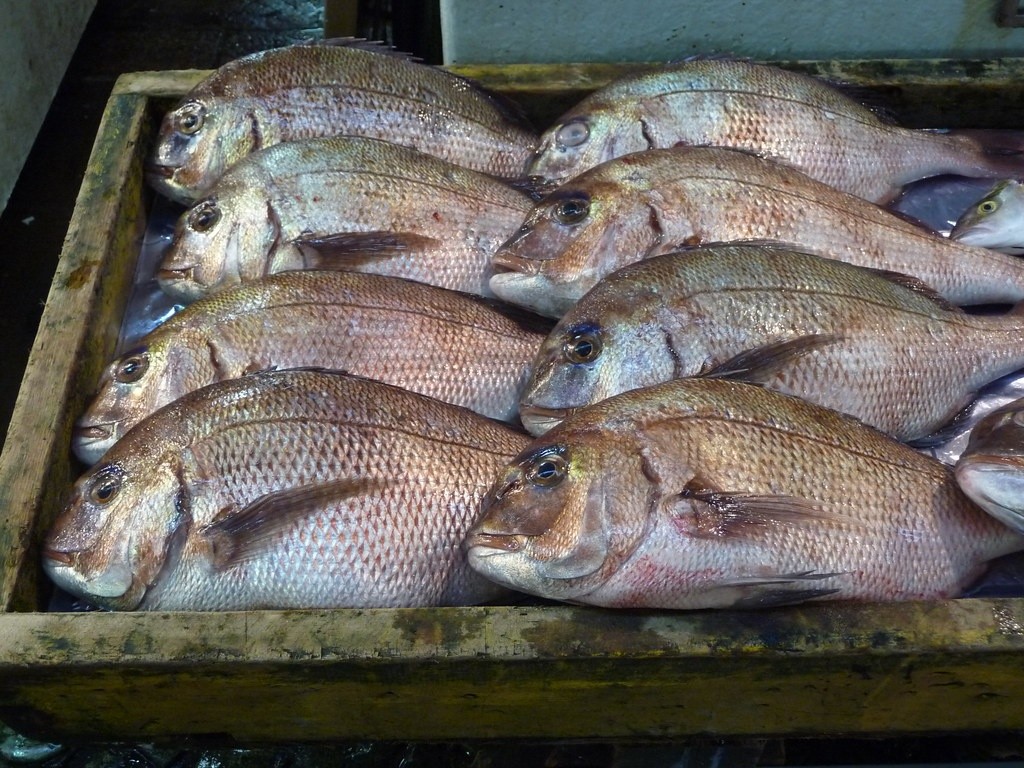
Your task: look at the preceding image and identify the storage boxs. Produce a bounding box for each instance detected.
[0,54,1024,731]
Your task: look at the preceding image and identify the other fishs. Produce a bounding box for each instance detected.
[35,39,1023,619]
